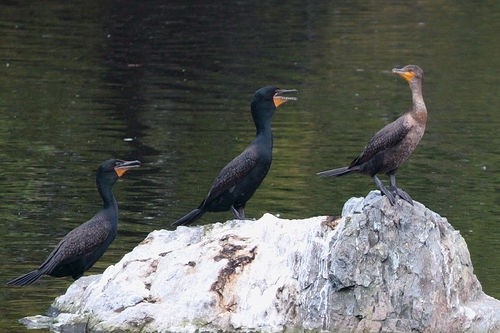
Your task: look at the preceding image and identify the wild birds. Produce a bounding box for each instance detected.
[170,87,298,228]
[315,64,428,206]
[3,159,142,288]
